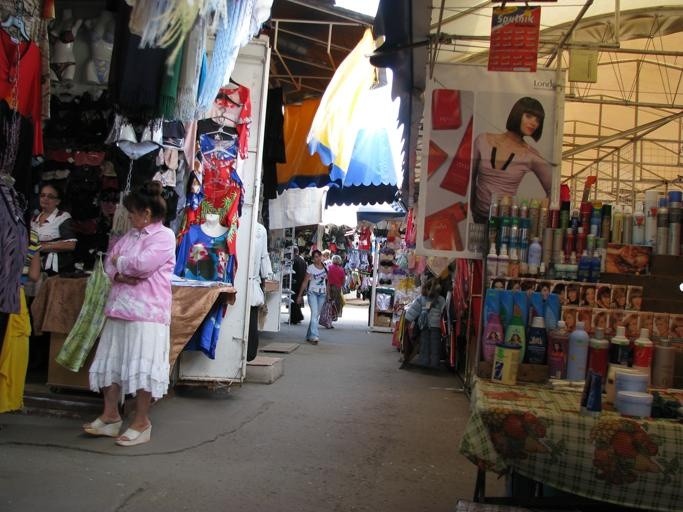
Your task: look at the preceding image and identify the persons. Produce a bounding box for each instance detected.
[490,277,641,310]
[552,342,563,356]
[46,7,82,83]
[509,333,522,345]
[292,246,345,346]
[173,213,233,358]
[82,11,116,88]
[486,331,500,342]
[562,307,682,340]
[470,91,554,226]
[80,178,176,448]
[0,181,120,355]
[358,221,407,249]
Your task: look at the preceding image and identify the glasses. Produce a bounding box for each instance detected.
[39,192,59,199]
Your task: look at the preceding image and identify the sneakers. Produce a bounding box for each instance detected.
[309,337,320,343]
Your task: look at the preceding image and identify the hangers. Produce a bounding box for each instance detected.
[198,69,245,170]
[0,10,30,43]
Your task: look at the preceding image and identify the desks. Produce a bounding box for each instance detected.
[461,368,682,511]
[28,270,236,398]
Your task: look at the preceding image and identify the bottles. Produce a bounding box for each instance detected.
[486,191,683,281]
[549,320,676,392]
[580,368,603,411]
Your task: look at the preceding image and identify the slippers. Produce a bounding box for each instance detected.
[114,424,152,448]
[82,417,123,437]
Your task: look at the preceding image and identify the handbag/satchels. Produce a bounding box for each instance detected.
[318,302,332,329]
[331,302,338,321]
